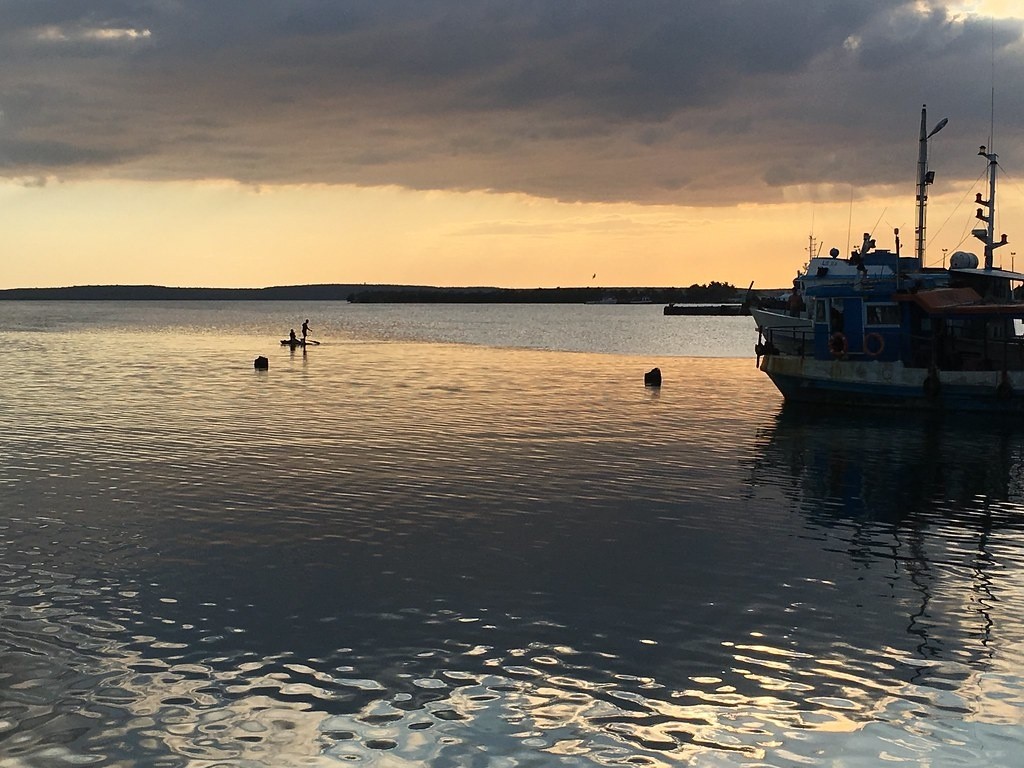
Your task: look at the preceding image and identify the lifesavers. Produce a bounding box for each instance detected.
[864,332,885,358]
[828,332,848,357]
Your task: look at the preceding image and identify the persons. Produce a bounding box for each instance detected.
[302,319,312,343]
[290,329,295,341]
[788,287,804,318]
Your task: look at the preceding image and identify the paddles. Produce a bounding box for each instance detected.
[298,337,320,345]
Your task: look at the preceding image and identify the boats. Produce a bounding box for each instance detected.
[282,337,316,346]
[749,103,1024,409]
